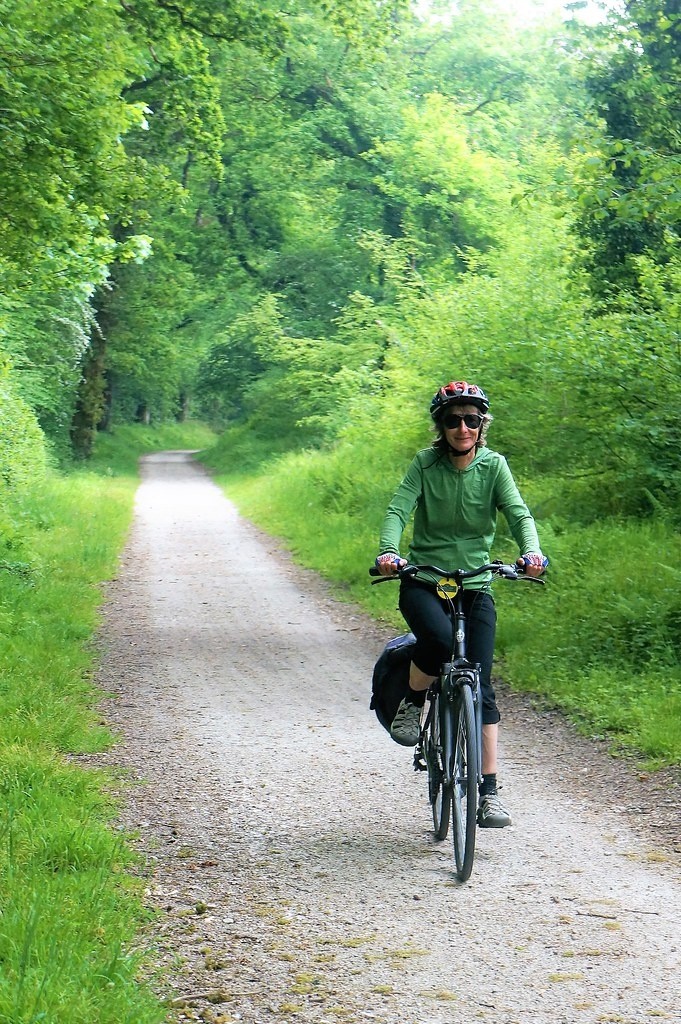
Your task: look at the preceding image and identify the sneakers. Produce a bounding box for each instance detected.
[391,697,420,747]
[478,794,511,828]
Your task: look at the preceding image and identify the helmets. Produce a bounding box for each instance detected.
[430,381,490,419]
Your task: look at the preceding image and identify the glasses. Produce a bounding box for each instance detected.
[440,414,485,429]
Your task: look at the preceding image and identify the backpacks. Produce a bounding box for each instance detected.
[369,633,417,732]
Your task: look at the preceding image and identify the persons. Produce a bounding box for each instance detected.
[375,379,550,829]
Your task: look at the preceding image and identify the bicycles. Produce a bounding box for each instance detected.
[369,558,547,883]
